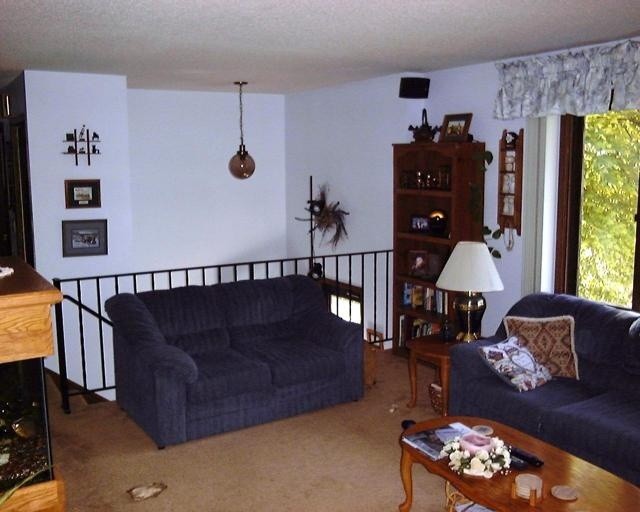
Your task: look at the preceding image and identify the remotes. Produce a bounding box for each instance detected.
[509,454,526,470]
[509,445,544,467]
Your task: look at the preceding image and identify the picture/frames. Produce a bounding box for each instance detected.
[63,179,103,210]
[438,112,474,142]
[61,218,108,259]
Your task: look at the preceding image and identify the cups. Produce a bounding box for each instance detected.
[436,165,451,185]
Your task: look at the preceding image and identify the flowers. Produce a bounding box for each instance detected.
[439,429,513,482]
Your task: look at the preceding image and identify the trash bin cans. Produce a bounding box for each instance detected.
[363,342,377,388]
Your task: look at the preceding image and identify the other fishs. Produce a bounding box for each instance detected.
[12,415,45,439]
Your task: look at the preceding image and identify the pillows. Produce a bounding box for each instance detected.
[502,314,581,382]
[476,335,553,394]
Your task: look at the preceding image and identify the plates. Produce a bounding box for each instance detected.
[550,485,578,500]
[515,473,543,498]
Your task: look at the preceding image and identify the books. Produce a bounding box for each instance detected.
[402,422,474,462]
[398,283,448,348]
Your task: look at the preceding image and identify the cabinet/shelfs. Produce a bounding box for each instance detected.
[392,142,486,368]
[0,254,69,512]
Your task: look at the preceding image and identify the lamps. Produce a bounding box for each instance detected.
[434,240,504,343]
[229,80,256,181]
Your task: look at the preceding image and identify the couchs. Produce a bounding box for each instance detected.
[104,273,366,450]
[446,290,640,489]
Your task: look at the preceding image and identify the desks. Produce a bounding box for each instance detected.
[405,334,452,419]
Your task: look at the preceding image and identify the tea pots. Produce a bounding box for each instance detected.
[408,107,439,142]
